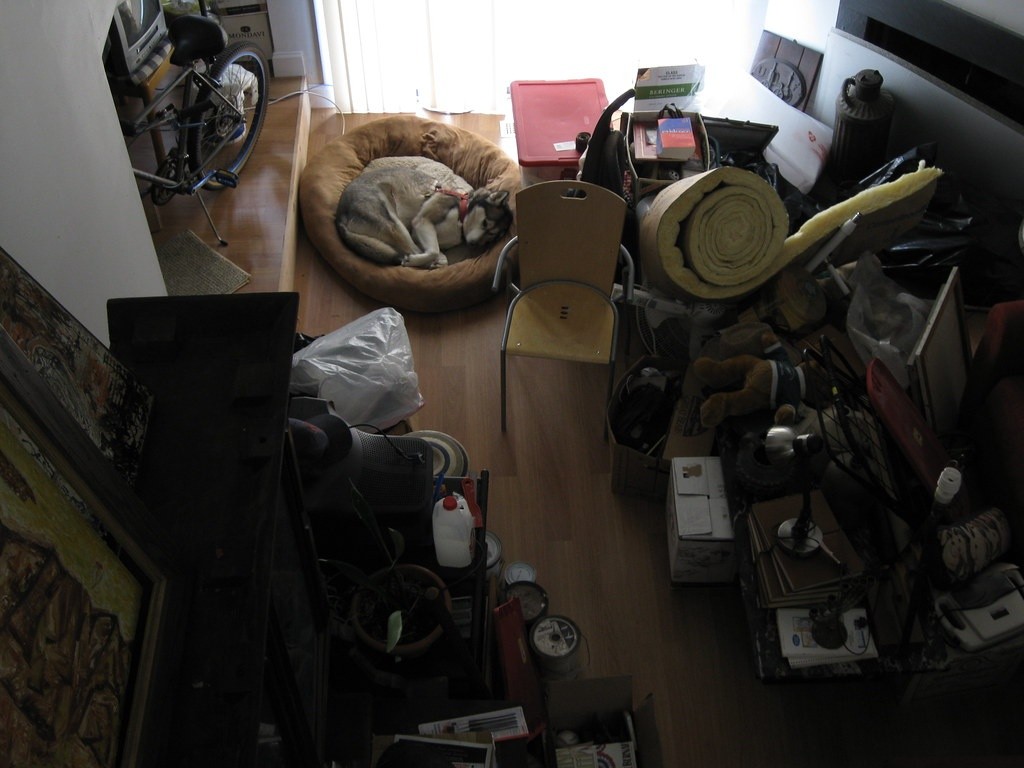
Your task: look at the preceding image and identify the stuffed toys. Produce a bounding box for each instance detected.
[693,355,828,427]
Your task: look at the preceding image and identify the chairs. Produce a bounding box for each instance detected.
[490,180,635,442]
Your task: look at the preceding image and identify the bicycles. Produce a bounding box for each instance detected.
[119,14,271,246]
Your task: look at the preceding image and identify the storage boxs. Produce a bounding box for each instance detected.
[541,675,640,768]
[626,111,711,206]
[510,78,609,191]
[666,456,736,590]
